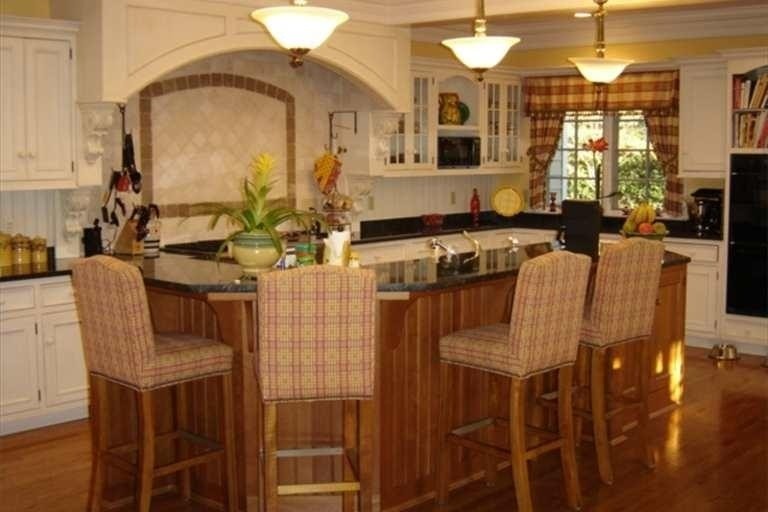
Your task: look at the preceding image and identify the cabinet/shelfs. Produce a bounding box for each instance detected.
[666,238,721,355]
[482,66,528,174]
[0,15,82,191]
[427,262,686,503]
[679,57,726,180]
[721,47,768,357]
[0,271,91,438]
[371,56,436,175]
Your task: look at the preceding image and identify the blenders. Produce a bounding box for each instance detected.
[692,188,723,237]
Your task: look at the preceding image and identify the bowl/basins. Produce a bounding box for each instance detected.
[421,214,443,225]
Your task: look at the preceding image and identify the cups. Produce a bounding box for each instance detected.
[82,227,102,257]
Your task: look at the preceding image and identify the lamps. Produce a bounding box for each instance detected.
[566,0,637,95]
[243,0,351,69]
[436,0,522,82]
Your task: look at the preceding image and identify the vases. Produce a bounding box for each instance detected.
[438,92,469,125]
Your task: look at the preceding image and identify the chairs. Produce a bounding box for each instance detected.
[66,253,237,511]
[437,250,593,511]
[531,235,668,485]
[257,265,383,510]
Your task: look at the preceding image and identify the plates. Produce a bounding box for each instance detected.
[492,187,524,216]
[619,230,670,241]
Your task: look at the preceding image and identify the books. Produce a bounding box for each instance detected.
[733,72,768,148]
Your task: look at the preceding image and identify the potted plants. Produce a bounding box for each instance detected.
[175,151,334,276]
[615,150,670,224]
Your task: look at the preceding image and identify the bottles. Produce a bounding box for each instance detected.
[0,231,12,266]
[470,189,480,216]
[12,232,32,264]
[145,222,160,257]
[32,235,48,263]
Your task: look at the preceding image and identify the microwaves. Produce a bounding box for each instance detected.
[439,137,481,168]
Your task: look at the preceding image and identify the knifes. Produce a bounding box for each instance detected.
[128,204,160,240]
